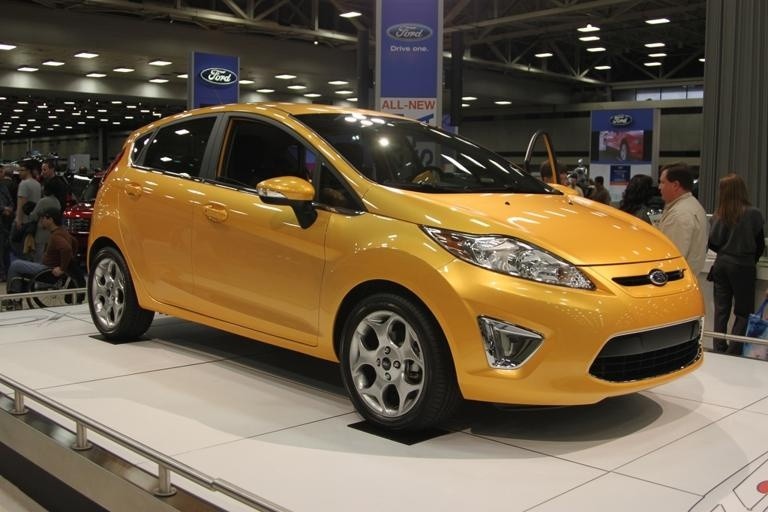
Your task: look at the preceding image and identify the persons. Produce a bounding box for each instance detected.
[560,158,612,205]
[708,170,764,355]
[656,162,710,277]
[0,159,79,311]
[620,174,655,225]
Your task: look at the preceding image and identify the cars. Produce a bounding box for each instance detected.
[84,102,708,440]
[61,169,110,260]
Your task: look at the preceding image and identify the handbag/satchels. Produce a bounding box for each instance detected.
[743,314,768,360]
[22,201,36,215]
[706,266,714,281]
